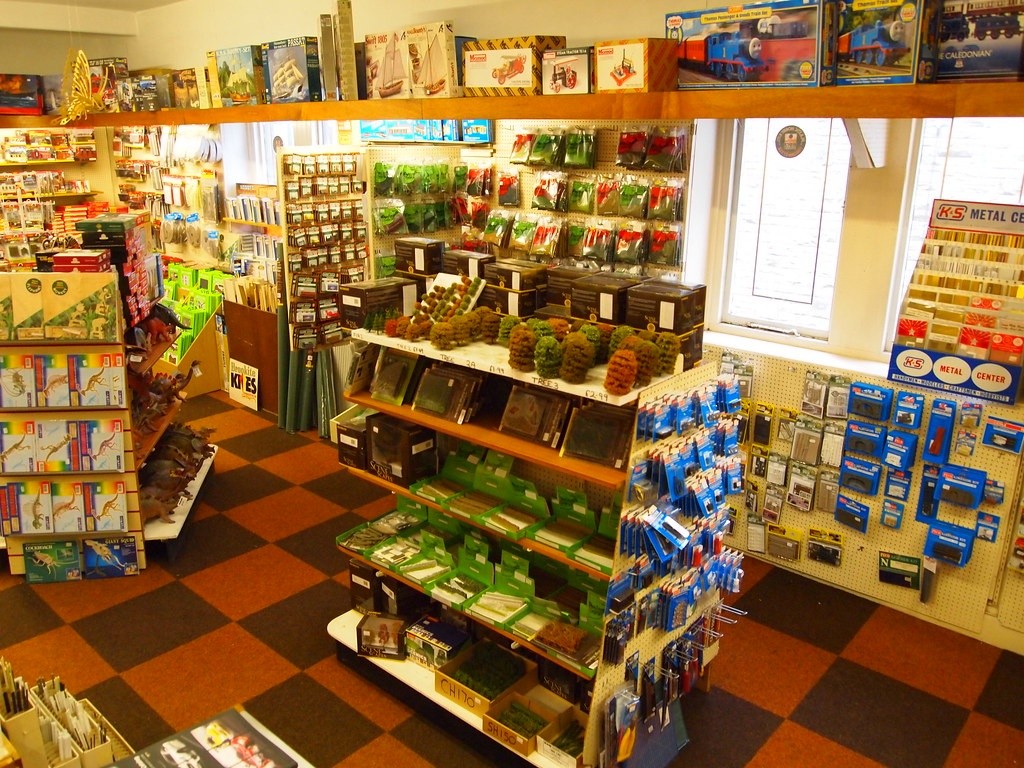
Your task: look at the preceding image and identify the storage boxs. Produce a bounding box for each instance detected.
[0,0,1024,117]
[34,201,165,328]
[339,237,707,372]
[349,558,590,768]
[336,423,368,473]
[364,410,439,489]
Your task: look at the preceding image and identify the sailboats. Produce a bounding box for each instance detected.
[373,32,406,98]
[414,30,448,94]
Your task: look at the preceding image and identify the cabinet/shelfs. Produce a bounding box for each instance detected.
[126,330,218,540]
[327,327,684,768]
[0,127,98,199]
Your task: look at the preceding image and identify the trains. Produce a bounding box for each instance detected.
[837,20,912,66]
[678,30,774,82]
